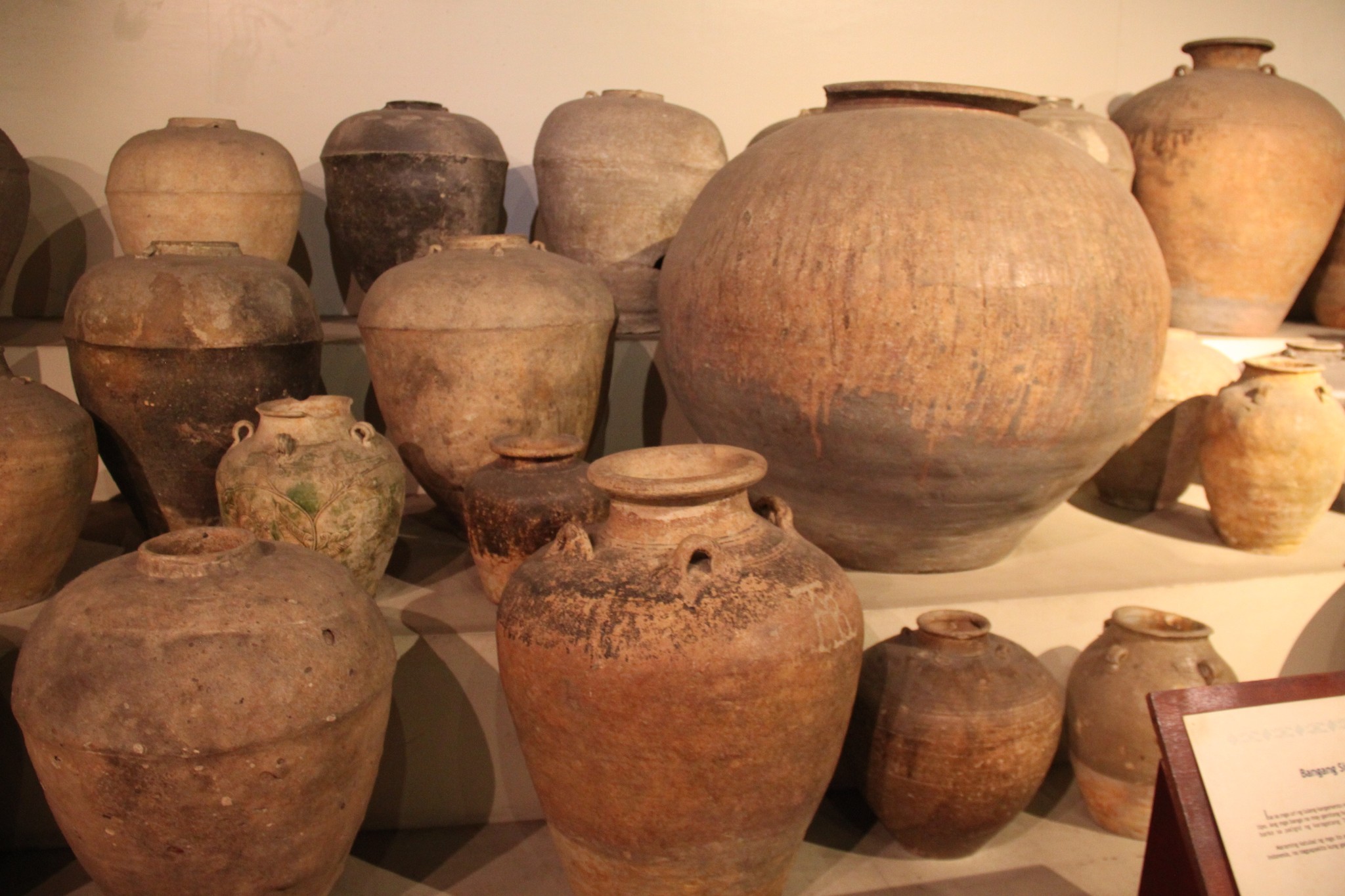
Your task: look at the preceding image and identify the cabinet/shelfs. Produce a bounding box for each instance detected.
[0,317,1345,895]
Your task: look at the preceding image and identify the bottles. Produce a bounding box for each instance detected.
[0,129,30,288]
[1090,327,1243,512]
[63,241,324,540]
[319,100,509,315]
[216,395,407,601]
[495,444,863,896]
[533,89,728,334]
[657,82,1171,574]
[847,610,1063,859]
[105,117,303,265]
[11,526,396,896]
[0,348,99,614]
[355,234,616,530]
[1266,339,1345,411]
[1110,37,1345,337]
[1065,606,1238,841]
[1197,356,1345,554]
[463,432,610,605]
